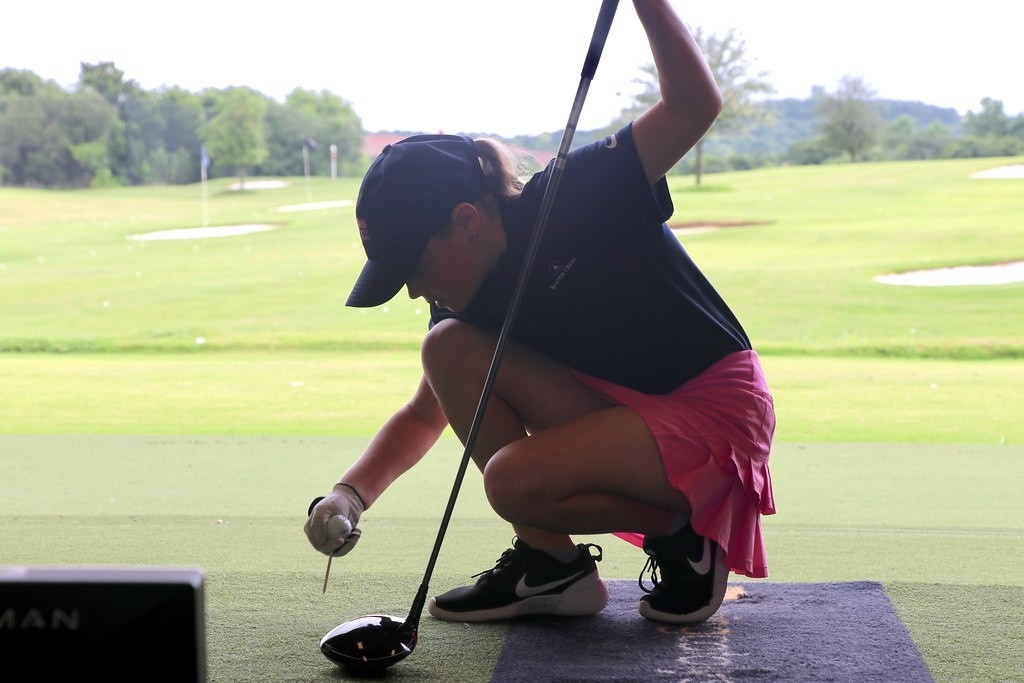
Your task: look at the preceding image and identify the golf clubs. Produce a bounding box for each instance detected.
[318,0,620,677]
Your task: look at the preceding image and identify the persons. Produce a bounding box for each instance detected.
[303,0,776,624]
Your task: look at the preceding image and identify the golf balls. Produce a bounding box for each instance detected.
[325,514,352,541]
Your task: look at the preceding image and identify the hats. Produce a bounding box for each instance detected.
[344,134,489,308]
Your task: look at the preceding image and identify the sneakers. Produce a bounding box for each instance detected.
[637,522,730,624]
[429,533,609,621]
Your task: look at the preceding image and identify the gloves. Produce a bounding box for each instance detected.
[303,483,365,558]
